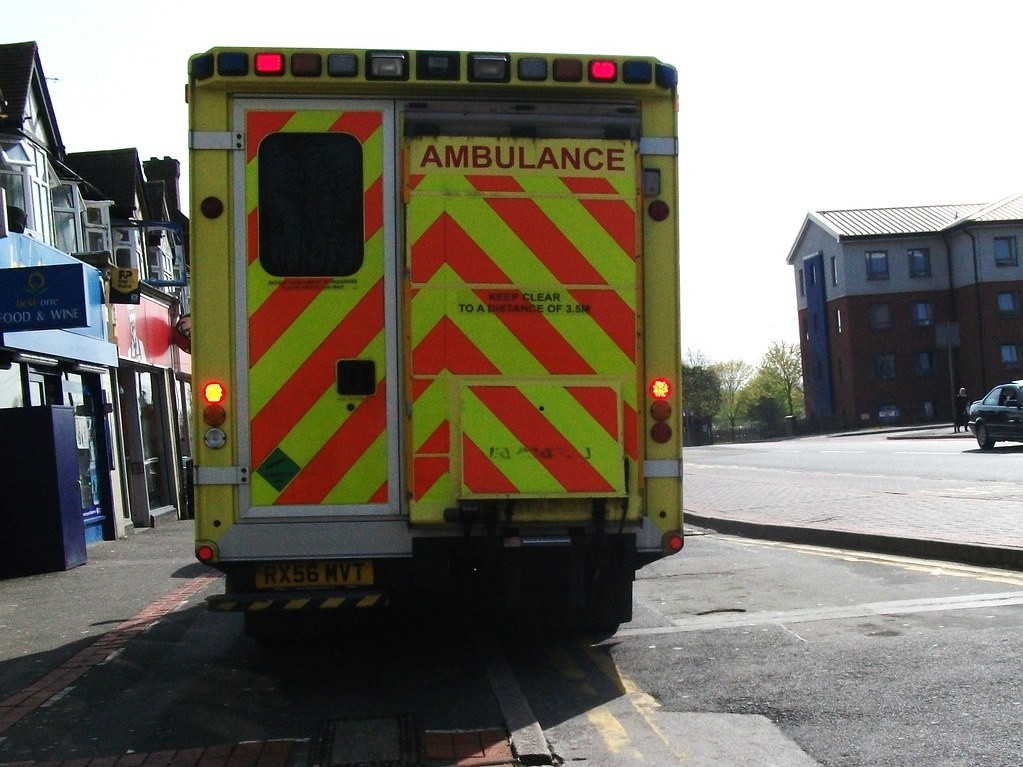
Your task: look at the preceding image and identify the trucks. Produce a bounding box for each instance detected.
[178,47,686,652]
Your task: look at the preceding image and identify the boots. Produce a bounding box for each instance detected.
[957,426,960,432]
[965,426,969,432]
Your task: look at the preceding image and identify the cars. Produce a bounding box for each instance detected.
[967,379,1023,450]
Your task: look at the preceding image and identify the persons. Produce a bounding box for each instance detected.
[956,388,971,433]
